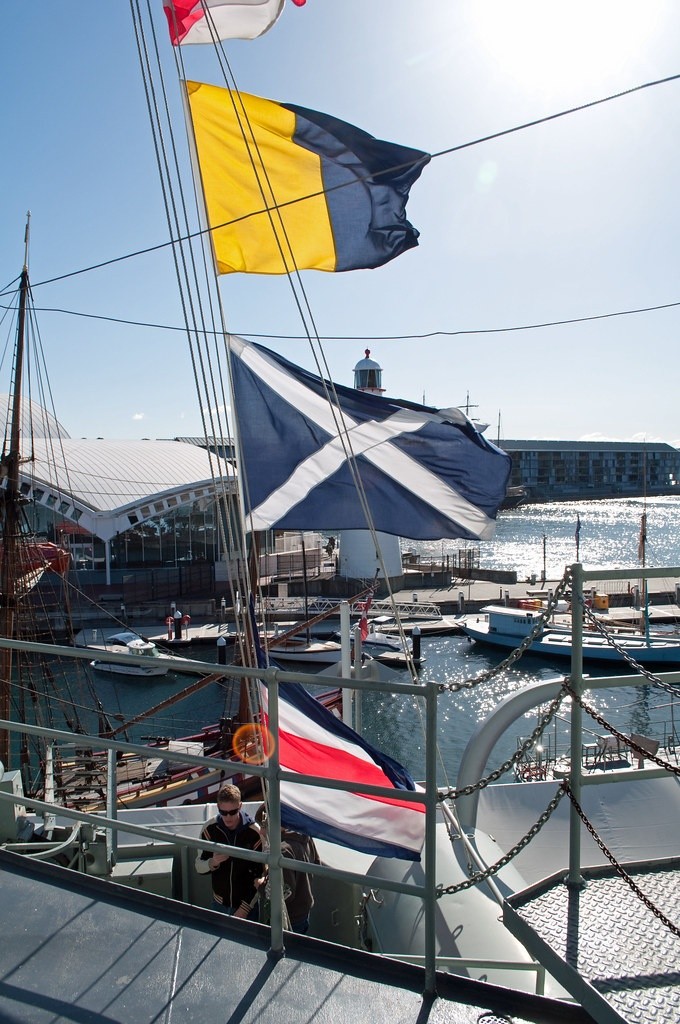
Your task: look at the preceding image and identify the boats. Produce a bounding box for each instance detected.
[90,645,172,674]
[259,636,341,662]
[456,603,680,665]
[19,684,357,810]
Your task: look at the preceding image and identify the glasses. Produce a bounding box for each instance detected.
[218,805,241,816]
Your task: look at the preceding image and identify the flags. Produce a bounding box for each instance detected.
[223,331,511,542]
[180,80,431,276]
[358,596,372,640]
[162,0,306,47]
[257,646,426,860]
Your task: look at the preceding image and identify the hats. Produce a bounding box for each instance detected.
[255,803,266,823]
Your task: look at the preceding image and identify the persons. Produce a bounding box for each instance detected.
[195,783,262,921]
[325,537,335,556]
[254,803,313,934]
